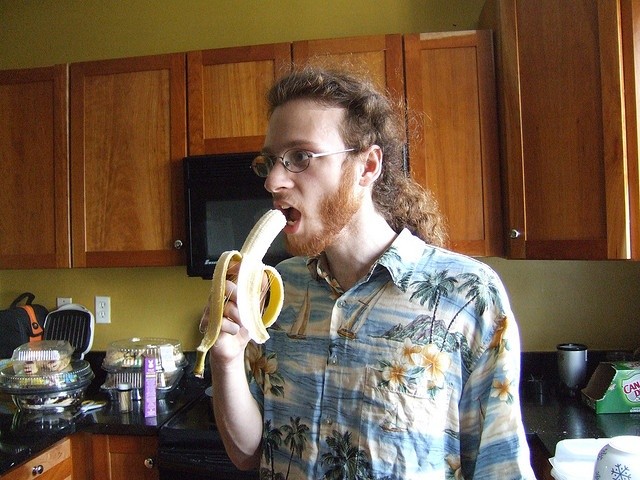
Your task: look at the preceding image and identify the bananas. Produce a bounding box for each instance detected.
[190,208,287,378]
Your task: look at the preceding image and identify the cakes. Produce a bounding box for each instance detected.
[103,336,185,368]
[13,391,82,409]
[8,371,85,388]
[11,338,74,375]
[103,369,178,388]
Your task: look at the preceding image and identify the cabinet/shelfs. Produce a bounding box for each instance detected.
[70,42,292,268]
[479,0,640,264]
[87,435,159,480]
[1,61,70,270]
[292,28,504,261]
[2,436,87,480]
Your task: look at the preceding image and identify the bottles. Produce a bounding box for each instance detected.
[118,383,133,413]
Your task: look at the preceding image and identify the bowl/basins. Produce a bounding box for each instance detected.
[596,436,639,479]
[12,379,89,414]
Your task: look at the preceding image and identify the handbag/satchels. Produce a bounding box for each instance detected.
[2,292,50,359]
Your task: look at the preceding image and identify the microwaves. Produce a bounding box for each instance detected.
[180,152,303,280]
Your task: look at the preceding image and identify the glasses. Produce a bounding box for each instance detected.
[249,147,356,177]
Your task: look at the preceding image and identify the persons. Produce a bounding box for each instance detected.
[200,71,539,480]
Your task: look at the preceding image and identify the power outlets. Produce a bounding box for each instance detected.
[95,296,112,324]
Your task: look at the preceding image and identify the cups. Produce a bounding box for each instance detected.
[558,344,588,394]
